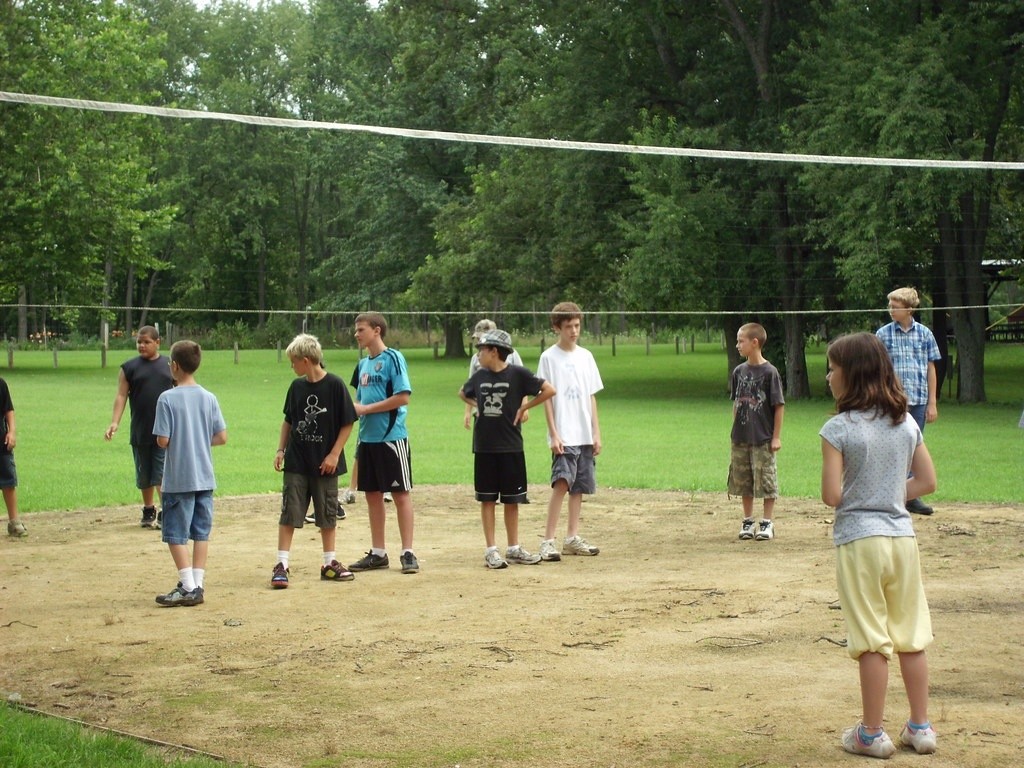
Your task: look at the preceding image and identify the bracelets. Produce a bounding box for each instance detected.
[277,449,284,452]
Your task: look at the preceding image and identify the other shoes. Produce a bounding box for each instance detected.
[336,503,346,519]
[305,513,315,522]
[338,492,355,503]
[906,498,933,515]
[383,491,393,502]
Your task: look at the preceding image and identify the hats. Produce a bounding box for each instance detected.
[475,330,513,353]
[472,319,497,339]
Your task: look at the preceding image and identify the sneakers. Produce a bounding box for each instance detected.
[562,536,599,556]
[321,560,354,581]
[155,586,195,606]
[8,521,28,538]
[755,520,774,539]
[485,549,509,569]
[540,542,561,561]
[195,587,204,603]
[401,552,418,573]
[842,723,894,758]
[739,517,756,539]
[349,549,388,570]
[271,562,289,586]
[899,722,936,753]
[156,512,164,529]
[141,505,157,527]
[506,547,542,564]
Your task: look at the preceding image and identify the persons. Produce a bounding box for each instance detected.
[103,325,177,530]
[305,502,346,522]
[875,286,941,515]
[345,362,393,504]
[0,379,28,537]
[463,319,528,429]
[536,302,605,561]
[271,334,359,589]
[726,323,785,539]
[348,312,420,574]
[152,340,227,604]
[821,336,938,756]
[458,329,557,569]
